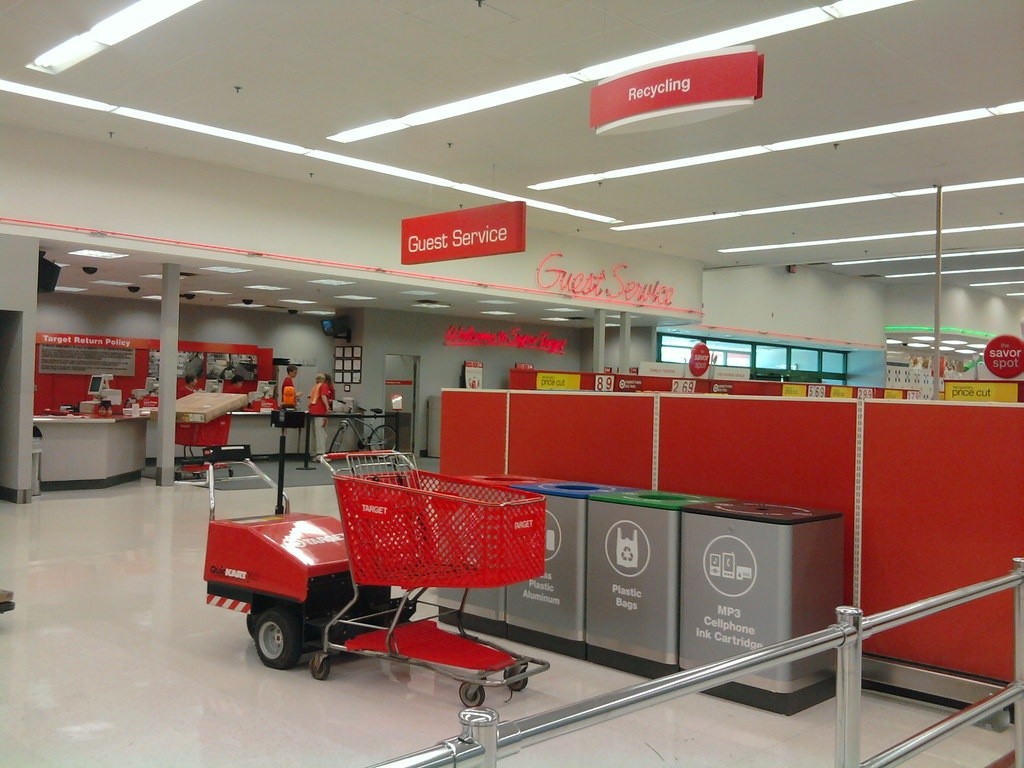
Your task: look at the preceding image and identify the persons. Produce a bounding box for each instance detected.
[177,373,198,399]
[219,353,239,379]
[308,373,333,462]
[325,374,335,411]
[282,365,303,408]
[223,375,246,394]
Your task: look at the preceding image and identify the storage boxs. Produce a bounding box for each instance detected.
[150,392,249,423]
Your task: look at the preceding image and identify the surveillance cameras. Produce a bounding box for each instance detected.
[40,251,46,256]
[84,267,299,315]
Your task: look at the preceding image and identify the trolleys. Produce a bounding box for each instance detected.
[309,447,552,707]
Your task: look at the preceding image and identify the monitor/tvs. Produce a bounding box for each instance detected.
[88,375,104,395]
[321,317,350,336]
[204,379,224,395]
[38,256,62,293]
[146,378,156,392]
[258,382,274,397]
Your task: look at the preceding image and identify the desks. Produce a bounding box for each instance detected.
[122,406,307,466]
[305,411,364,458]
[33,412,150,491]
